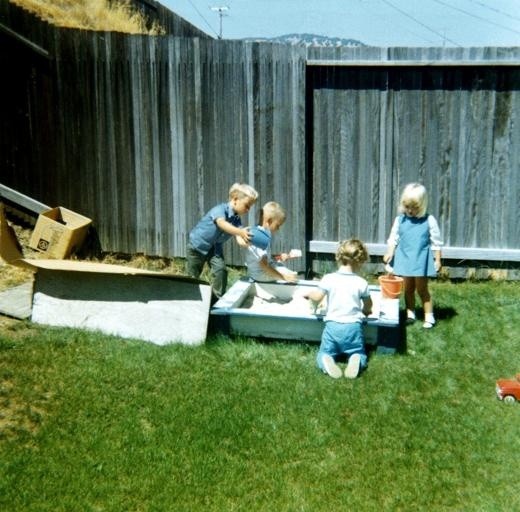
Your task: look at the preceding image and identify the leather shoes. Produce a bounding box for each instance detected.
[422,320,437,330]
[405,317,416,326]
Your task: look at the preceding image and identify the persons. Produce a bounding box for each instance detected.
[382,182,444,328]
[240,201,298,285]
[187,182,259,301]
[301,239,374,379]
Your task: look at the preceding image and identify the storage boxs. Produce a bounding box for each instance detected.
[30,206,92,261]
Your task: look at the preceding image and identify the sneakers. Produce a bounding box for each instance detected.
[322,355,342,379]
[344,353,361,379]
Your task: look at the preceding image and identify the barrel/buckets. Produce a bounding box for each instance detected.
[378,276,405,299]
